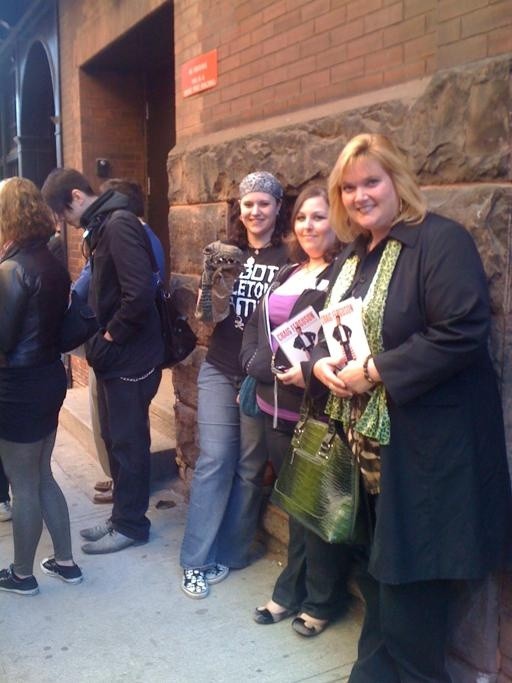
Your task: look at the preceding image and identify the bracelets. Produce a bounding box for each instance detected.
[363,354,383,386]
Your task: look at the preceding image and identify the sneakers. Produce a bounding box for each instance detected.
[37,557,83,587]
[0,502,13,521]
[203,558,231,584]
[0,562,43,596]
[179,560,208,602]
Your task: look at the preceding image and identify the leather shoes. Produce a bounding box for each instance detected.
[81,529,150,554]
[81,513,115,539]
[92,479,118,505]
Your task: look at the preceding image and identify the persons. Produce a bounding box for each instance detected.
[293,327,315,353]
[180,171,297,599]
[1,167,166,594]
[240,180,348,637]
[304,132,512,682]
[332,316,353,362]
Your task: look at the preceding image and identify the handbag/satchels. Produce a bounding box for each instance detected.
[271,355,363,545]
[154,289,197,370]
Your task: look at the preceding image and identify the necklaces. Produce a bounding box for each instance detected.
[307,262,326,273]
[248,241,271,255]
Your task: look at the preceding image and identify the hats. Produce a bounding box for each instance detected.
[235,172,285,202]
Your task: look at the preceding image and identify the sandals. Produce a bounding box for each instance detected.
[291,608,331,636]
[254,597,299,624]
